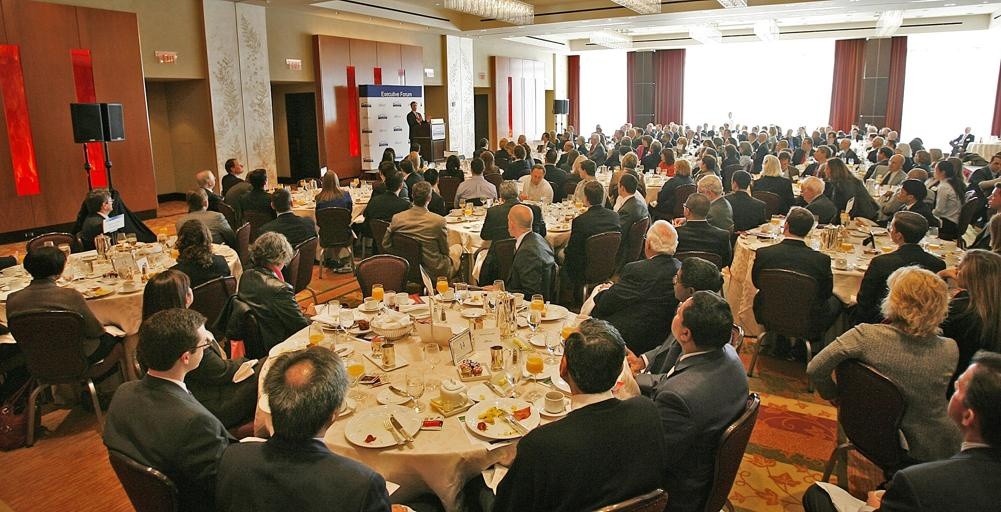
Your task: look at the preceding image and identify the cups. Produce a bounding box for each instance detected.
[422,160,471,174]
[381,344,396,368]
[864,178,900,198]
[543,391,565,413]
[489,346,505,371]
[512,292,525,306]
[439,377,468,410]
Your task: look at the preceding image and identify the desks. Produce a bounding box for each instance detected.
[253,291,641,511]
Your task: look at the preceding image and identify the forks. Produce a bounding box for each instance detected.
[497,408,528,436]
[383,419,405,446]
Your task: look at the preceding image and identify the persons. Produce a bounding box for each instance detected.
[314,113,1001,463]
[651,290,750,512]
[855,347,999,512]
[213,347,410,512]
[5,159,315,413]
[406,101,422,154]
[102,309,240,512]
[475,318,664,510]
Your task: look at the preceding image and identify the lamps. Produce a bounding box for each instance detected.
[586,29,635,56]
[713,0,752,9]
[441,0,537,25]
[604,1,663,17]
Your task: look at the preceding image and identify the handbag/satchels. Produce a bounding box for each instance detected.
[0,400,39,449]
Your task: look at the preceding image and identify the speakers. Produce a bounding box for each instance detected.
[99,103,126,142]
[70,103,106,142]
[554,100,569,114]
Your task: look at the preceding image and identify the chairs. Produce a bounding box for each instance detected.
[7,307,130,445]
[15,141,936,307]
[822,357,909,495]
[705,393,760,509]
[746,267,820,393]
[188,275,236,358]
[602,489,670,509]
[106,449,182,512]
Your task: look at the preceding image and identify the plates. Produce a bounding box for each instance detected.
[462,293,488,305]
[258,393,273,414]
[334,343,354,357]
[444,194,586,233]
[341,396,357,417]
[539,397,572,416]
[523,363,554,379]
[275,178,373,209]
[465,397,540,440]
[0,226,179,302]
[467,385,502,399]
[460,308,487,318]
[551,372,572,394]
[539,303,569,322]
[344,403,423,450]
[365,349,409,372]
[377,386,412,404]
[757,210,960,273]
[530,332,559,347]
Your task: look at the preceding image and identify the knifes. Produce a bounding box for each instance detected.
[388,413,415,443]
[389,385,409,397]
[483,381,505,398]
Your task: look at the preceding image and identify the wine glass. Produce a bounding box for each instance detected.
[424,343,442,380]
[595,164,668,187]
[841,157,856,172]
[544,330,561,357]
[531,294,544,319]
[405,370,425,413]
[292,277,508,348]
[525,352,545,384]
[790,177,807,195]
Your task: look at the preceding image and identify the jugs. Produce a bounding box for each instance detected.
[495,292,517,339]
